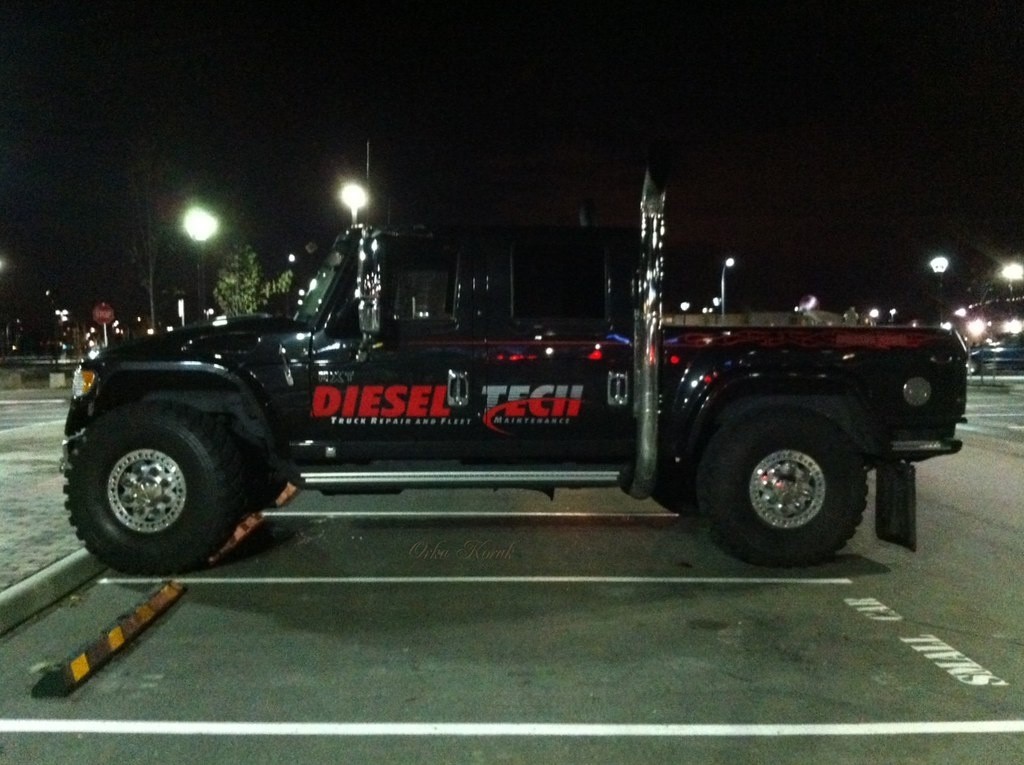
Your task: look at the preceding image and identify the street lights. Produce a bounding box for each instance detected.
[720,258,734,320]
[931,256,949,323]
[341,185,368,228]
[186,210,217,319]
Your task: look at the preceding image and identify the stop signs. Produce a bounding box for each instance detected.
[92,302,114,325]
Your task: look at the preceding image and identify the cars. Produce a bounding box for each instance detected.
[946,296,1024,376]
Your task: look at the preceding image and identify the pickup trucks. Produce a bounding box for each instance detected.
[59,159,969,579]
